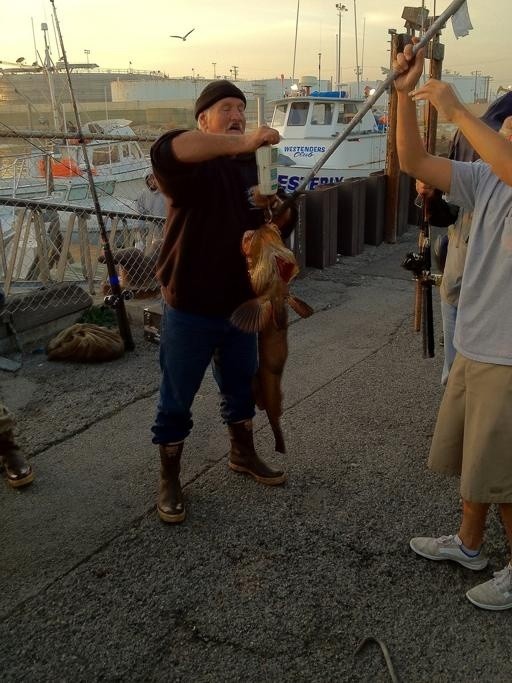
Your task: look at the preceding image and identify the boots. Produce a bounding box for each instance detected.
[227,417,288,485]
[156,439,187,523]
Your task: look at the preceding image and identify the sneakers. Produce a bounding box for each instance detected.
[409,533,487,572]
[466,562,511,611]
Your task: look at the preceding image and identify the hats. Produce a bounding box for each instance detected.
[194,79,247,122]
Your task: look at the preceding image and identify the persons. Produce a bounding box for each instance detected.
[134,167,168,246]
[413,89,511,387]
[147,79,287,523]
[389,36,511,612]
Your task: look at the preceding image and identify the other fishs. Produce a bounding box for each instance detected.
[230,222,314,454]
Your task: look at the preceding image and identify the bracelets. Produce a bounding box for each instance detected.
[268,191,281,213]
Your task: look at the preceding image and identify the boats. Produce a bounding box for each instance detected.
[1,22,158,247]
[269,1,387,191]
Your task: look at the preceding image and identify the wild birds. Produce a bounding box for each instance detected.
[169,29,195,41]
